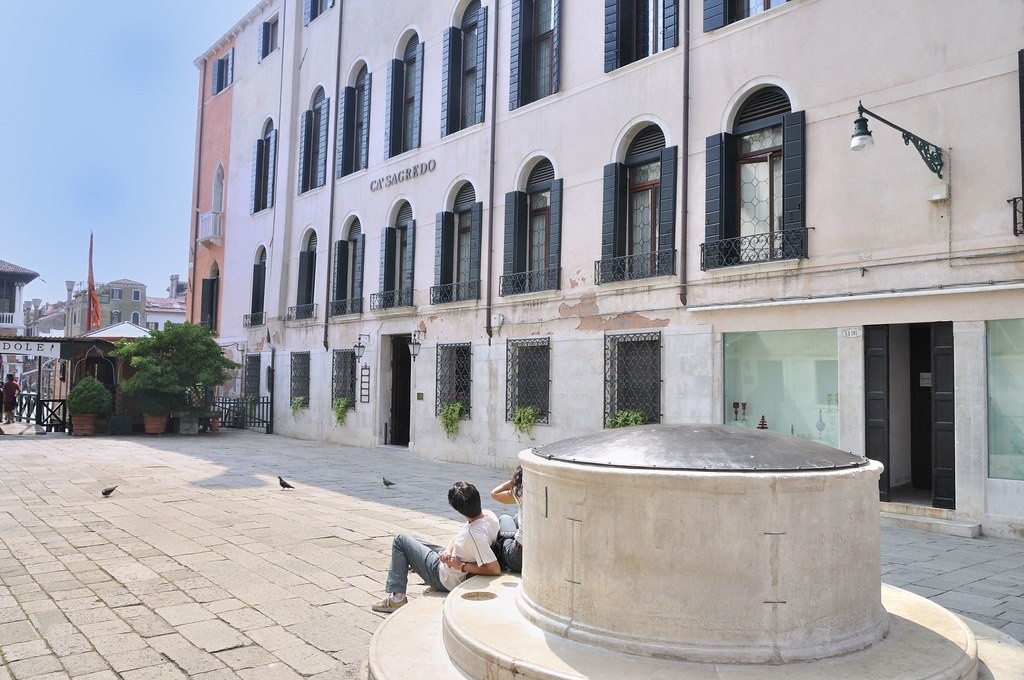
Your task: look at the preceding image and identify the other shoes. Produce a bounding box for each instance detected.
[12,421,15,423]
[4,421,10,424]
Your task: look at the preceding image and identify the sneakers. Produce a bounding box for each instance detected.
[372,594,407,612]
[423,584,436,595]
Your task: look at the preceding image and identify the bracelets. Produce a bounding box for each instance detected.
[461,562,468,573]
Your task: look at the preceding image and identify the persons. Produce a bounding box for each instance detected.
[0,373,30,424]
[372,481,501,613]
[491,466,523,576]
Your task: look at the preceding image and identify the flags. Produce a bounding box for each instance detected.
[86,233,101,330]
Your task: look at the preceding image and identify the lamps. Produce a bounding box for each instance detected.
[850,100,943,179]
[353,333,371,363]
[408,329,427,362]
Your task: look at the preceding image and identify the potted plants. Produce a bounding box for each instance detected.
[66,376,113,436]
[135,389,174,434]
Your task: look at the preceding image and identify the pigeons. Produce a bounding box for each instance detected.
[277,476,295,490]
[382,477,396,488]
[101,485,118,497]
